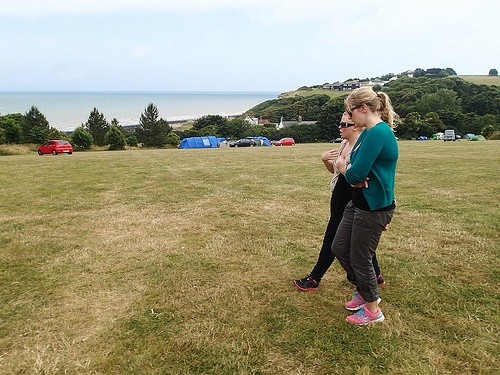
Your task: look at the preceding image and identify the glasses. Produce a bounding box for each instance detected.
[340,122,355,128]
[347,105,363,117]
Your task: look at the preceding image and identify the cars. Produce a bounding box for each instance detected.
[275,138,296,146]
[38,140,73,155]
[331,138,342,143]
[229,139,256,147]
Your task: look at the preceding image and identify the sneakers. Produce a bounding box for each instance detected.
[290,276,318,290]
[377,274,385,288]
[346,306,385,325]
[345,292,382,310]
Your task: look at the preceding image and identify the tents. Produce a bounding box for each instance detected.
[178,135,272,149]
[417,132,486,141]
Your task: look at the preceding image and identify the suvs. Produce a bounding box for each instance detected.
[443,130,455,142]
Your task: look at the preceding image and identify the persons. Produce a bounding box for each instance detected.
[331,86,399,325]
[292,110,387,290]
